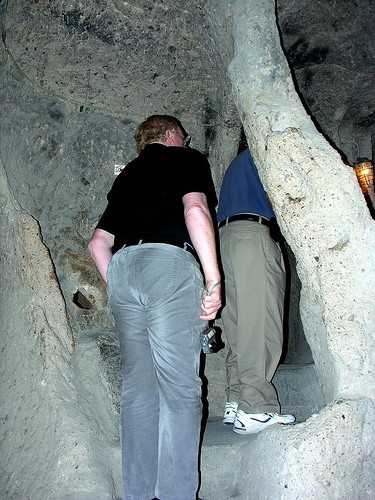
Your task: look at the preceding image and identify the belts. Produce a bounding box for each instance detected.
[111,239,200,263]
[218,215,269,227]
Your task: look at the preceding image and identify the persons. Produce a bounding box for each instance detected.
[88,115,223,500]
[217,148,296,436]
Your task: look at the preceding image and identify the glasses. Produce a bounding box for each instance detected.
[164,128,191,146]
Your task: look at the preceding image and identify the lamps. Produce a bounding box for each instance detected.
[353,157,375,210]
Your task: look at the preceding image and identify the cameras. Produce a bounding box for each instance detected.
[200,327,219,354]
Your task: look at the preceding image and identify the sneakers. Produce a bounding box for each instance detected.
[223,401,239,423]
[233,410,295,435]
